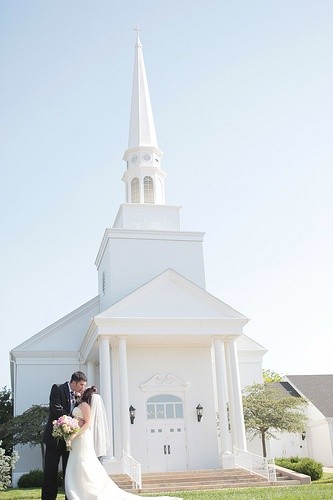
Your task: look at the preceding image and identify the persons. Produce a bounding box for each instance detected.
[63,385,184,500]
[41,371,85,500]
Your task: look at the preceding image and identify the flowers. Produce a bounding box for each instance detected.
[51,414,81,452]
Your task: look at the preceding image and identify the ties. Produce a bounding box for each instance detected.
[70,391,75,412]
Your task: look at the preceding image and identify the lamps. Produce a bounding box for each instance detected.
[129,405,136,424]
[196,404,203,422]
